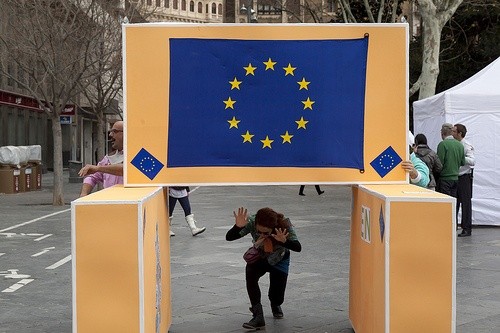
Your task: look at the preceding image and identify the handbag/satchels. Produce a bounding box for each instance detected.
[242,247,262,264]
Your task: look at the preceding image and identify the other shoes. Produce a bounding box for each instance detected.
[299,193,305,196]
[319,191,324,195]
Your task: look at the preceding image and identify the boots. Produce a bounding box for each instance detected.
[169,216,175,236]
[184,213,206,236]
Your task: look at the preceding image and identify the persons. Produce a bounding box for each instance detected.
[299,185,324,196]
[226,206,302,330]
[169,186,206,236]
[78,121,123,197]
[401,123,476,237]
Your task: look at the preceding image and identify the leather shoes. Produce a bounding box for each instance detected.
[242,317,265,329]
[458,230,471,236]
[270,305,283,318]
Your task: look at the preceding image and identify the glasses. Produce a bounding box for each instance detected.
[109,129,123,133]
[255,230,271,236]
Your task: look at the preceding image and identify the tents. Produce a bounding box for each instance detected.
[412,57,500,226]
